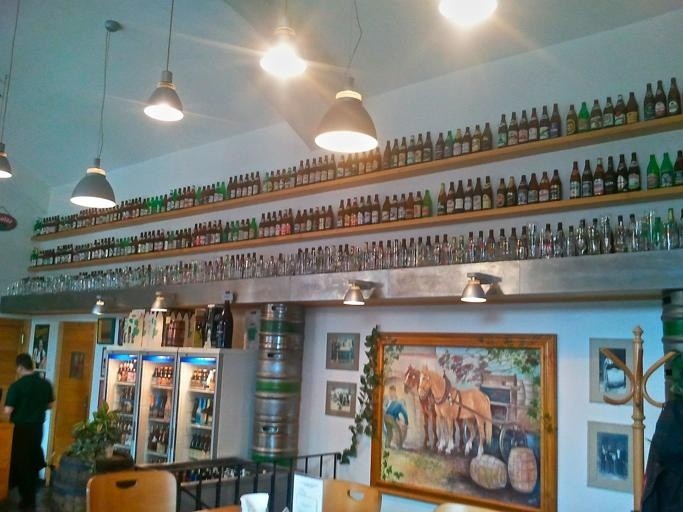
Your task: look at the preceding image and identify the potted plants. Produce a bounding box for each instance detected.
[71,397,127,460]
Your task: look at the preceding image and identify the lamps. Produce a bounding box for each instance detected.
[0,2,27,181]
[69,19,123,212]
[150,288,171,314]
[139,1,188,123]
[255,1,308,82]
[458,272,508,309]
[313,0,380,157]
[343,280,382,309]
[92,295,107,318]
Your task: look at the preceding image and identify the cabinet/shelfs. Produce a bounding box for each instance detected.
[25,114,682,275]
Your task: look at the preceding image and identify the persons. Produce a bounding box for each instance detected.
[385,386,396,411]
[4,353,56,510]
[34,336,46,370]
[384,399,409,450]
[599,442,625,478]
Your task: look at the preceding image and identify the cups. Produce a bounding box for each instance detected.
[240,492,269,511]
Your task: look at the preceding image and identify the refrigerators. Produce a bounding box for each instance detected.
[101,349,253,467]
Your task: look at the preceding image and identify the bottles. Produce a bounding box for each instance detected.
[6,77,682,294]
[204,299,233,349]
[147,365,173,464]
[187,367,214,454]
[172,463,245,482]
[114,359,136,445]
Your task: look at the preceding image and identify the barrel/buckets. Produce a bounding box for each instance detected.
[47,449,136,511]
[469,455,507,491]
[506,379,525,404]
[661,287,683,402]
[505,448,538,494]
[251,303,305,468]
[522,380,534,407]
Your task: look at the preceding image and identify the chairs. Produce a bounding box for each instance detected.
[84,463,178,512]
[321,475,383,512]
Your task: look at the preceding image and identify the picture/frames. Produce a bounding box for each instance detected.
[32,322,50,380]
[588,333,636,408]
[326,332,359,372]
[325,380,357,418]
[585,417,633,494]
[369,331,560,512]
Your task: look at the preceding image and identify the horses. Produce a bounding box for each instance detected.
[402,364,465,451]
[417,363,492,458]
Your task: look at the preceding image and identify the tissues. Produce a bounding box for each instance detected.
[238,491,271,512]
